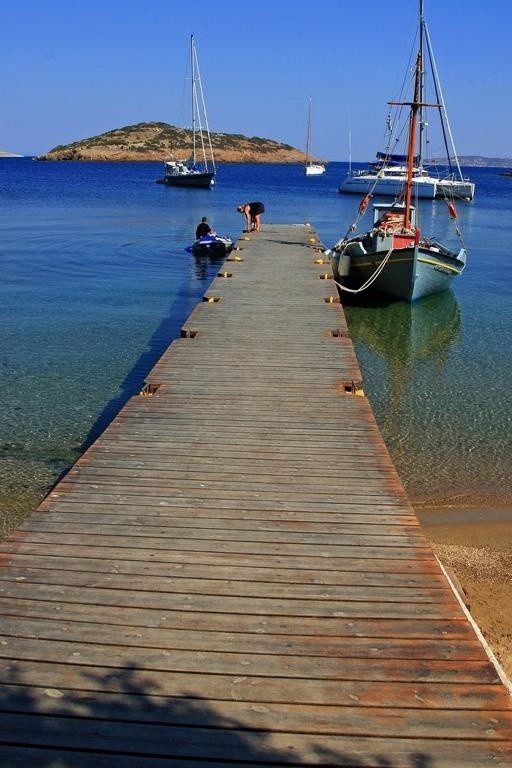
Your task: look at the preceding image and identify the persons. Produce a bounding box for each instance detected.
[196,216,216,239]
[236,201,265,233]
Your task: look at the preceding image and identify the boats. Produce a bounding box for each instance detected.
[187,236,237,256]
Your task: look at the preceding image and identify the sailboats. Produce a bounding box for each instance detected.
[333,0,472,307]
[334,0,477,203]
[151,29,218,191]
[303,95,328,176]
